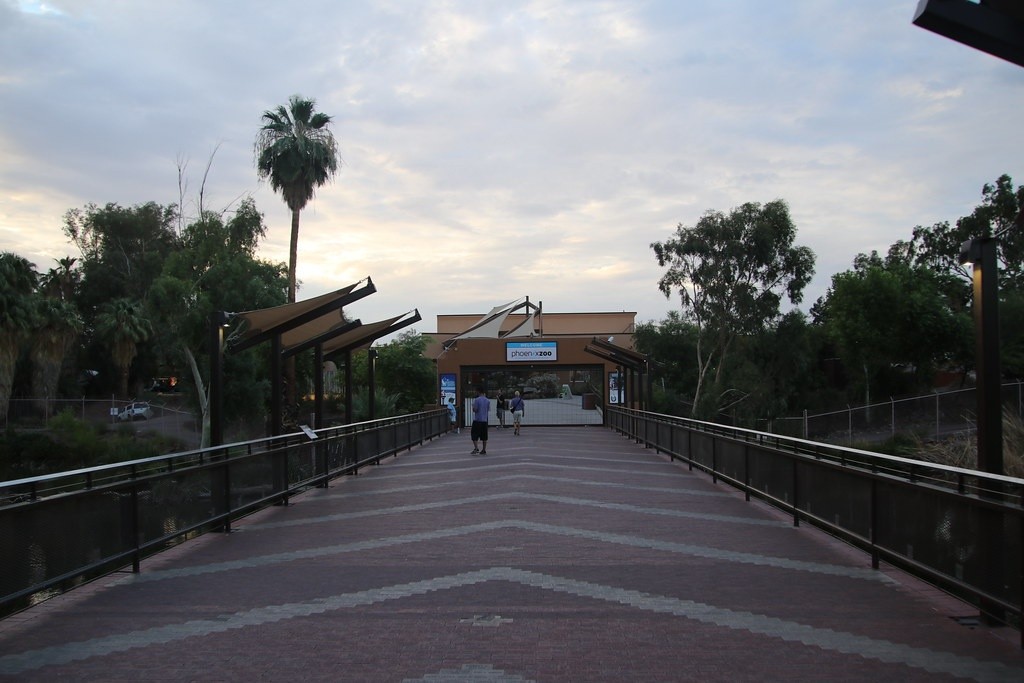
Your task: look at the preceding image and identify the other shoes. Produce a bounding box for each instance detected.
[514,429,519,436]
[471,448,479,455]
[480,450,486,455]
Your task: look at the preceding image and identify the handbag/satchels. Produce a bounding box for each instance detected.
[510,408,514,414]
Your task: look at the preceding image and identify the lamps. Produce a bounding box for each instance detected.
[607,336,614,343]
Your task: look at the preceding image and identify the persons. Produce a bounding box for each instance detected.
[509,391,525,436]
[496,388,504,430]
[446,397,456,432]
[470,387,490,456]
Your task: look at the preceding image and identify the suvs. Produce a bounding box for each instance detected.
[117,402,151,423]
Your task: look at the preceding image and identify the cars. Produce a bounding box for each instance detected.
[145,376,177,393]
